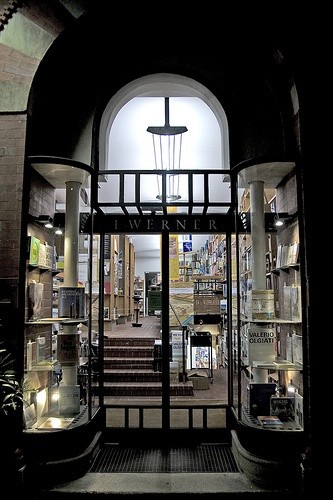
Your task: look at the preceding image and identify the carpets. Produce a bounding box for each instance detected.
[88,444,241,473]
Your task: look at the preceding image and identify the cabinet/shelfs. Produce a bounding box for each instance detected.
[178,154,309,490]
[17,155,137,485]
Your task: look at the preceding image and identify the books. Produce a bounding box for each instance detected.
[15,229,305,430]
